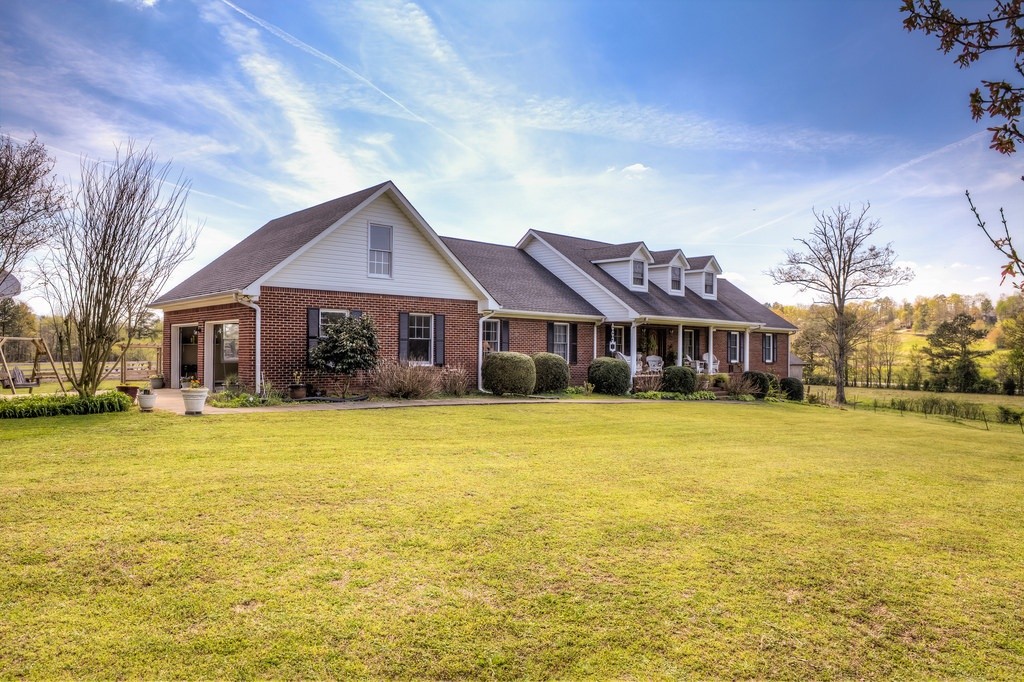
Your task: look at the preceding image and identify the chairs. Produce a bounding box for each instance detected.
[682,353,721,375]
[616,351,664,375]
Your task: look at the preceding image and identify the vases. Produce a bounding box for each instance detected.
[181,387,210,414]
[180,383,190,388]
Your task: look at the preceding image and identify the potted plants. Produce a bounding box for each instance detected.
[116,373,164,412]
[287,371,307,399]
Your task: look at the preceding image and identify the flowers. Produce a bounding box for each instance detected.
[180,374,202,388]
